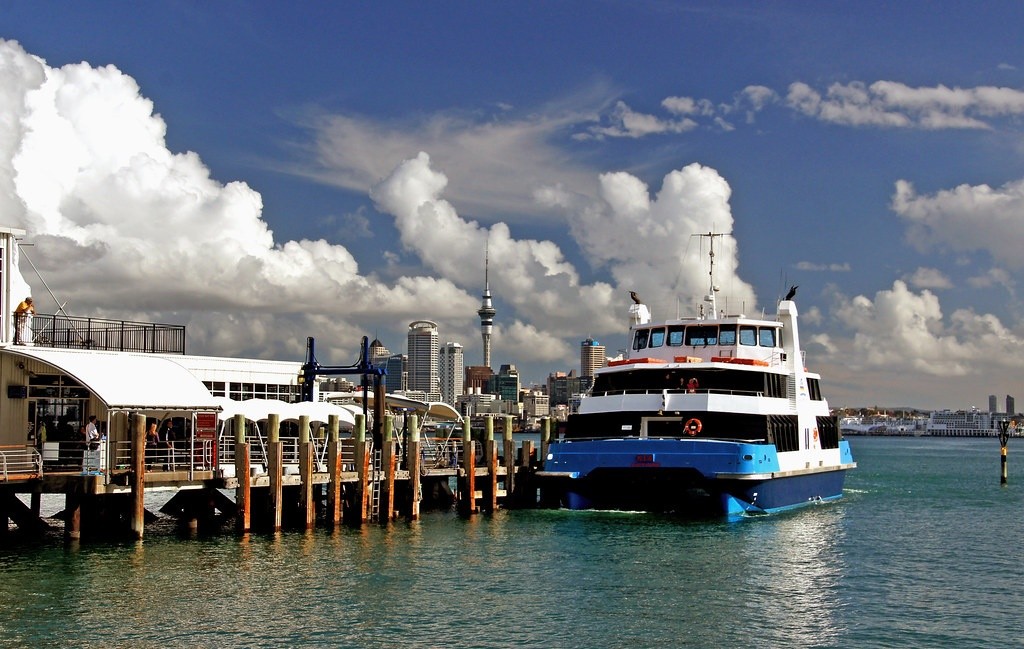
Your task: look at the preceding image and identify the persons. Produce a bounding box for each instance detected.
[13,298,35,345]
[145,424,159,473]
[657,373,674,393]
[686,378,700,393]
[37,419,47,471]
[160,421,177,471]
[79,415,100,471]
[674,377,686,392]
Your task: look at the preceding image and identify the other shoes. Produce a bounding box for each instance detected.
[13,341,26,346]
[146,469,153,472]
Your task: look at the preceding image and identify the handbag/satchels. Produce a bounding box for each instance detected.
[89,438,100,450]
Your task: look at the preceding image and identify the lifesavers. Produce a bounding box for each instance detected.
[684,417,702,436]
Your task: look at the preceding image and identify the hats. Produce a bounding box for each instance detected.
[26,297,33,301]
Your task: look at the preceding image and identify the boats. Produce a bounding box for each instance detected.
[531,222,858,525]
